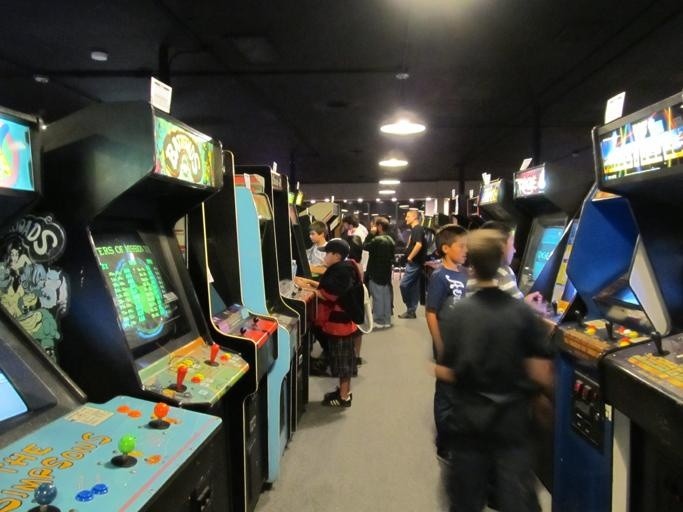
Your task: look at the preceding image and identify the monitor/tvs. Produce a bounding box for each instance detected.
[92,223,184,356]
[208,269,232,321]
[528,221,563,284]
[1,369,30,426]
[609,282,643,309]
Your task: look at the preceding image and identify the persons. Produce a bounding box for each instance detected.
[303,220,333,267]
[327,206,429,330]
[471,218,548,324]
[429,228,557,512]
[425,224,469,465]
[293,238,364,408]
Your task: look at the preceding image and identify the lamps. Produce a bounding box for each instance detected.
[375,66,426,197]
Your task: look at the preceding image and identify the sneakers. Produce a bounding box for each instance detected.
[322,396,351,407]
[376,324,390,328]
[399,311,416,319]
[325,387,352,401]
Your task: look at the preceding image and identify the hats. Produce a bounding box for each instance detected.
[317,237,351,258]
